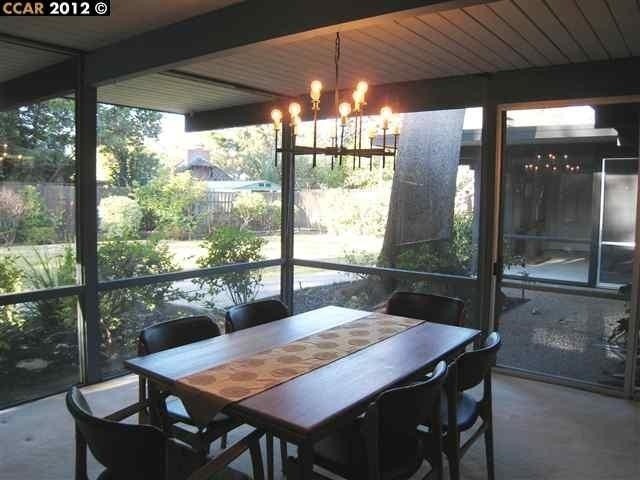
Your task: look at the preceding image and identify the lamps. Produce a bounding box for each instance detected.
[269,32,401,167]
